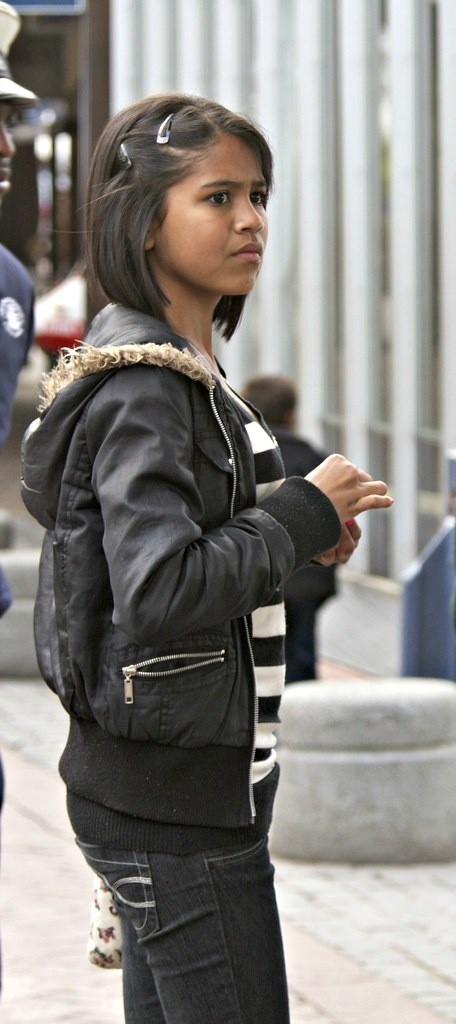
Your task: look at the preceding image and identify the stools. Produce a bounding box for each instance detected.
[270,674,456,865]
[0,548,41,681]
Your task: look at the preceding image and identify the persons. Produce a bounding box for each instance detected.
[240,373,338,684]
[21,90,394,1023]
[0,0,34,815]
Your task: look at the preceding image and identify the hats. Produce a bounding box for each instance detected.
[0,1,39,110]
